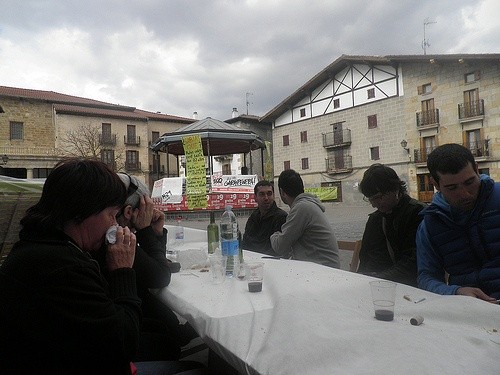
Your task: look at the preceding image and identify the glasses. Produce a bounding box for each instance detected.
[363,194,385,203]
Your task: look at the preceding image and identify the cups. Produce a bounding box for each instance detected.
[233,255,249,281]
[208,256,227,283]
[244,263,264,292]
[369,281,397,320]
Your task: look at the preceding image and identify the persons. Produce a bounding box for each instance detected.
[356,163,428,289]
[270,169,341,269]
[239,180,289,259]
[0,157,214,375]
[416,143,500,306]
[95,171,196,362]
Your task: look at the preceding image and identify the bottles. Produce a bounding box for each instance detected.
[237,223,243,263]
[207,212,219,254]
[175,220,184,244]
[219,206,238,279]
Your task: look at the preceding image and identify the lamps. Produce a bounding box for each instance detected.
[401,139,409,154]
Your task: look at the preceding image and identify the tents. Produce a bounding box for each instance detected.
[149,117,266,194]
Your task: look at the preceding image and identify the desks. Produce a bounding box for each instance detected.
[153,225,500,375]
